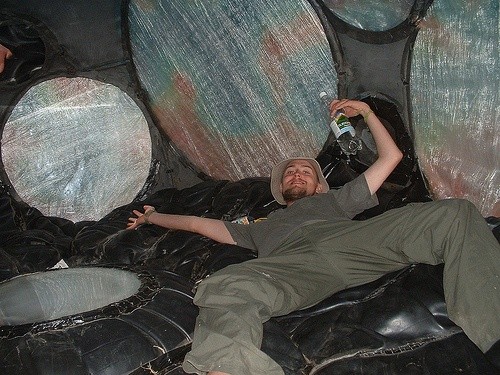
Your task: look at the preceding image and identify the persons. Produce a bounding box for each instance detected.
[125,99,500,375]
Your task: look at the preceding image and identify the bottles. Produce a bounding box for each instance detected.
[320,91,363,155]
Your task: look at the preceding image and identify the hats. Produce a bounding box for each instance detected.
[271,158,330,206]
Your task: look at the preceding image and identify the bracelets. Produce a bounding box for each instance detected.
[364,109,373,123]
[144,210,156,225]
